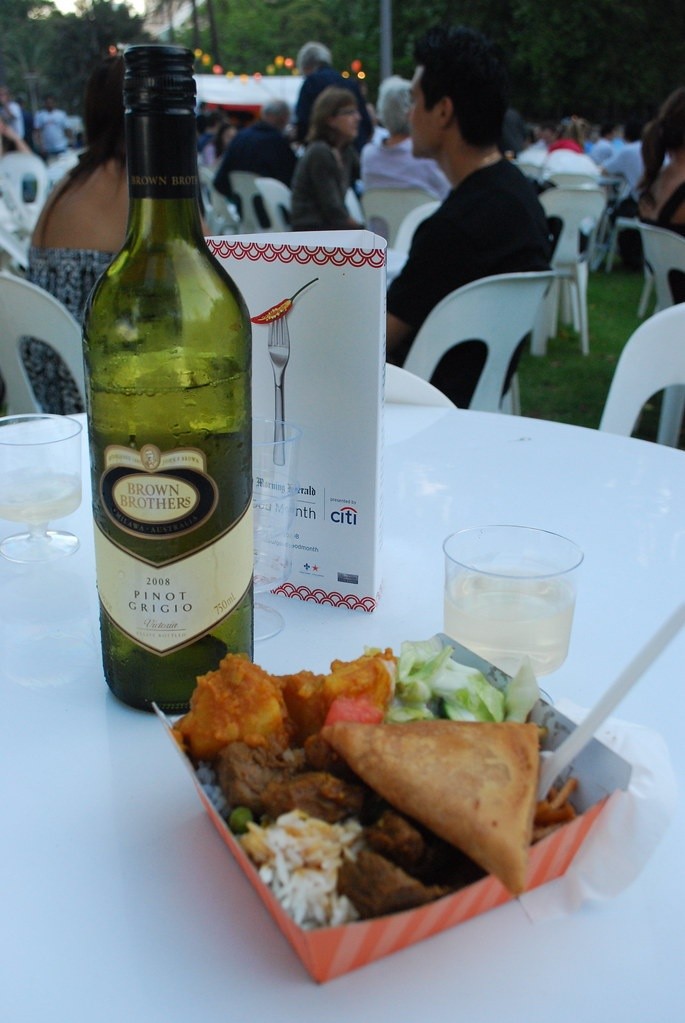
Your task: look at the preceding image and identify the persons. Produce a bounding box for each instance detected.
[15,55,141,414]
[378,22,553,412]
[498,88,685,278]
[198,43,451,237]
[0,84,86,172]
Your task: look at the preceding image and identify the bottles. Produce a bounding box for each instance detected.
[81,45,258,714]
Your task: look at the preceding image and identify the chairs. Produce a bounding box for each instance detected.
[0,150,685,448]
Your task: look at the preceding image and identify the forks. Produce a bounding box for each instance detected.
[268,314,291,465]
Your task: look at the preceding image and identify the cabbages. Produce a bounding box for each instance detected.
[383,638,541,724]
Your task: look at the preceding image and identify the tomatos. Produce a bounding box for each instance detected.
[325,696,382,726]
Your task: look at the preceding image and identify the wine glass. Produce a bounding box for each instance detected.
[442,526,585,712]
[1,415,84,566]
[253,415,302,646]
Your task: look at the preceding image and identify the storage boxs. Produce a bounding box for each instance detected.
[153,633,633,986]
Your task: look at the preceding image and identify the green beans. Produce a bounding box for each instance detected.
[229,806,252,833]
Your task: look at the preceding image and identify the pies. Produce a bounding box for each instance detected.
[315,719,542,898]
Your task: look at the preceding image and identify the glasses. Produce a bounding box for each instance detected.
[337,108,360,115]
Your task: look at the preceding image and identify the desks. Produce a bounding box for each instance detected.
[0,406,685,1023]
